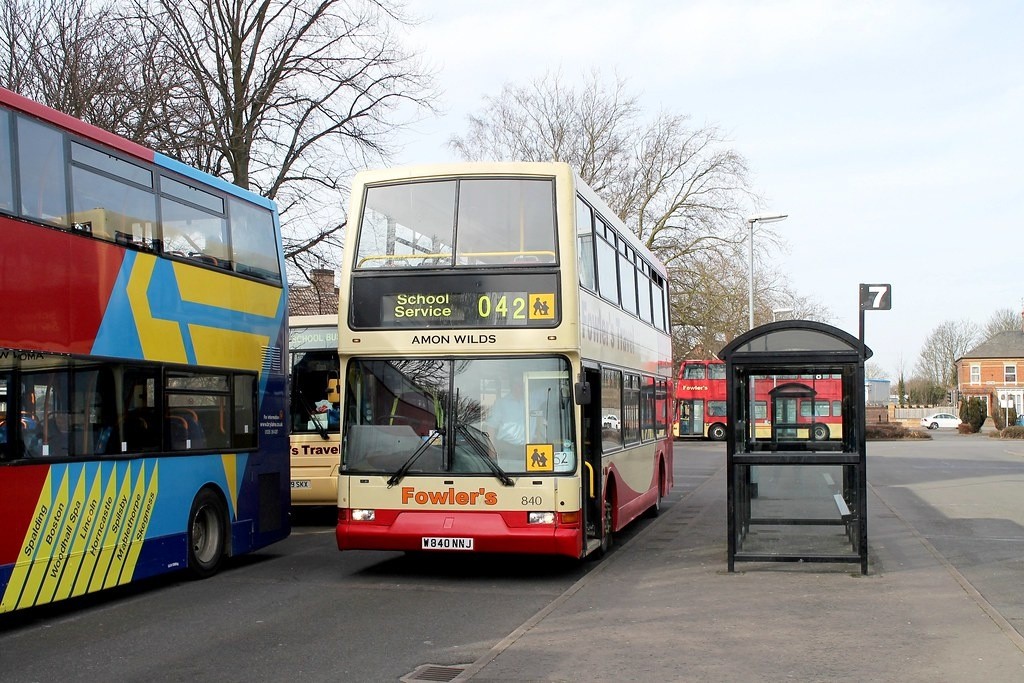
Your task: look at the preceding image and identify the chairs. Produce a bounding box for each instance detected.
[134,241,217,268]
[0,407,205,455]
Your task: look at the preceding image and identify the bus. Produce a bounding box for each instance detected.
[337,162,843,559]
[234,314,445,505]
[673,360,842,441]
[0,87,290,615]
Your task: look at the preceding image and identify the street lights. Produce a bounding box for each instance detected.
[747,213,788,442]
[773,308,793,388]
[546,388,551,443]
[895,390,896,398]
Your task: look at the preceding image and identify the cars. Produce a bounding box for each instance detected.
[602,414,620,430]
[920,413,962,429]
